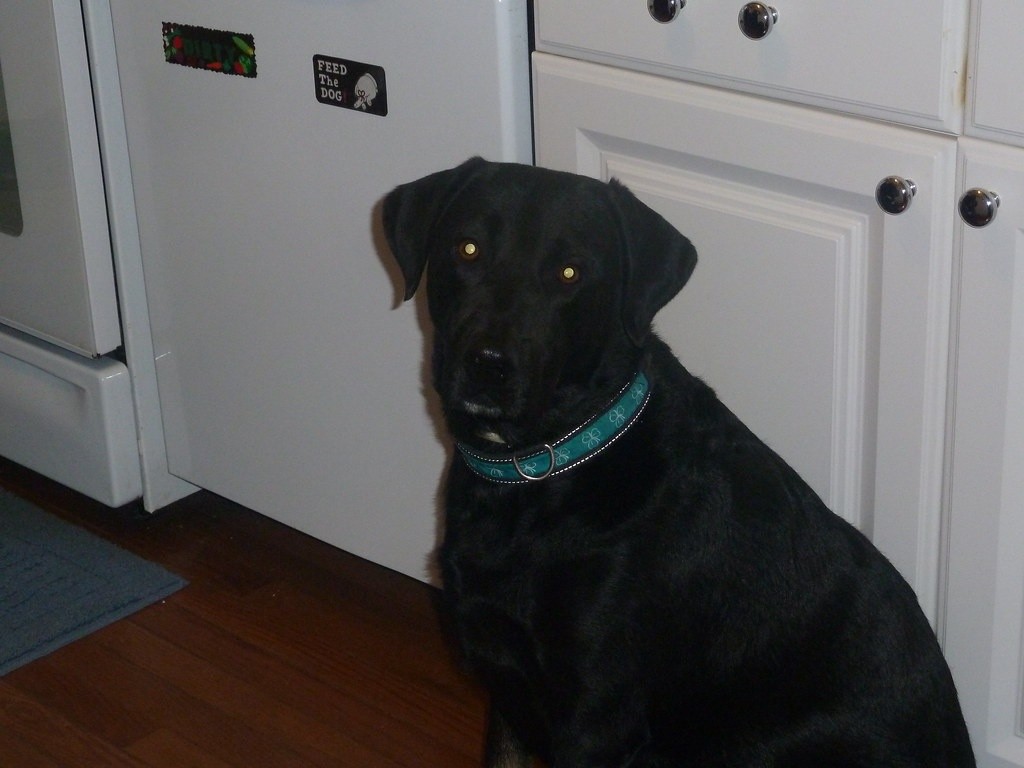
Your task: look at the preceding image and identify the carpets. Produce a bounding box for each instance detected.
[0,485,191,674]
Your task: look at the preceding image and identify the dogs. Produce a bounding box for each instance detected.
[382,156,977,767]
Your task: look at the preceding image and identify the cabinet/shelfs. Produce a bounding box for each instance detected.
[532,2,1023,767]
